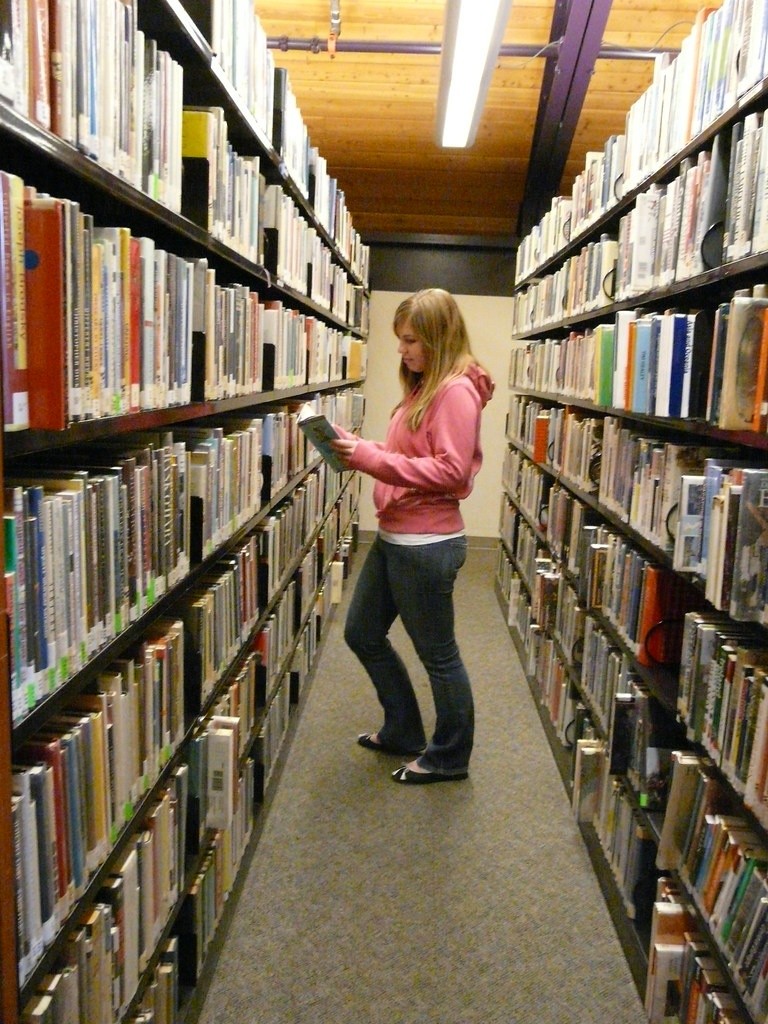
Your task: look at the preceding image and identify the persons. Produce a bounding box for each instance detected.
[327,288,495,784]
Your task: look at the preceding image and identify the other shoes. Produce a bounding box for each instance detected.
[393,764,468,784]
[358,733,428,755]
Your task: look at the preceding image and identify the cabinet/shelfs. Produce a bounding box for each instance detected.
[0,0,372,1024]
[499,83,767,1023]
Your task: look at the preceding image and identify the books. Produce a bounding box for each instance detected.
[501,322,615,611]
[185,256,366,403]
[295,403,355,474]
[511,133,626,336]
[610,283,768,433]
[3,432,191,724]
[494,491,632,788]
[621,0,768,197]
[586,529,768,830]
[181,1,368,336]
[205,472,358,952]
[598,416,768,627]
[0,0,184,215]
[572,617,768,1024]
[161,461,355,717]
[614,109,768,302]
[11,619,203,1023]
[0,170,192,431]
[168,385,365,565]
